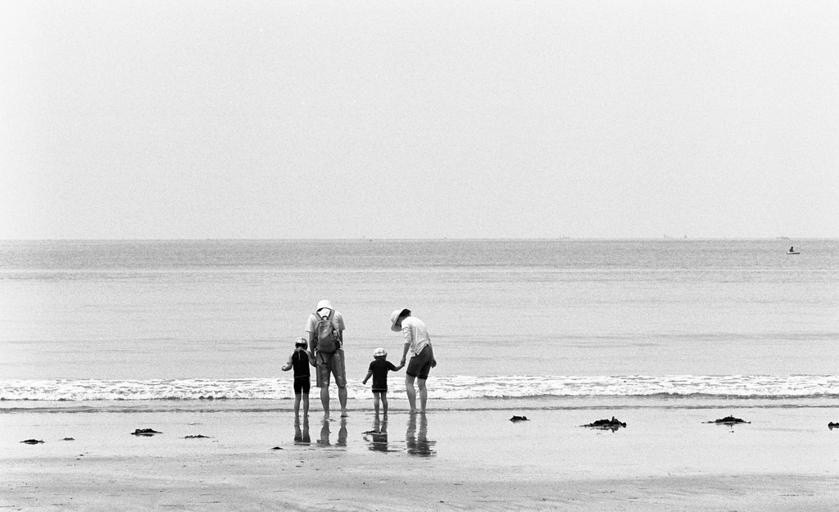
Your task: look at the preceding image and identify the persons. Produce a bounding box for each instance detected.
[282,299,348,445]
[362,308,436,414]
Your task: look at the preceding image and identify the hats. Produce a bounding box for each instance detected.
[373,348,387,359]
[317,300,333,311]
[295,337,308,349]
[390,308,412,332]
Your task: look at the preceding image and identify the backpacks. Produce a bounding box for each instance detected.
[312,308,340,354]
